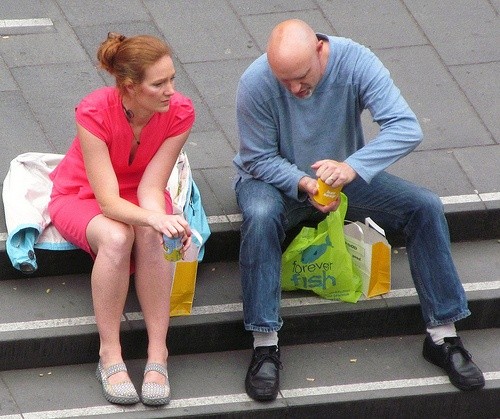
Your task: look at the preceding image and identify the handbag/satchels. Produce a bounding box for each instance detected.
[343,216,391,297]
[168,228,202,317]
[280,192,363,303]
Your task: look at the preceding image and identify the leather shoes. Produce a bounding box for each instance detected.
[245,343,283,400]
[423,335,485,391]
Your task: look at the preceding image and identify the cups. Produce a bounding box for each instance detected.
[312,177,343,206]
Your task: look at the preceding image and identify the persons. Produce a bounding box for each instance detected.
[232,18,486,403]
[48,31,196,407]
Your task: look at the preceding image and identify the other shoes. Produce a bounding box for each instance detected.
[141,364,171,406]
[95,359,139,404]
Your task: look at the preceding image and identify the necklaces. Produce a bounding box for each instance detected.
[122,103,155,145]
